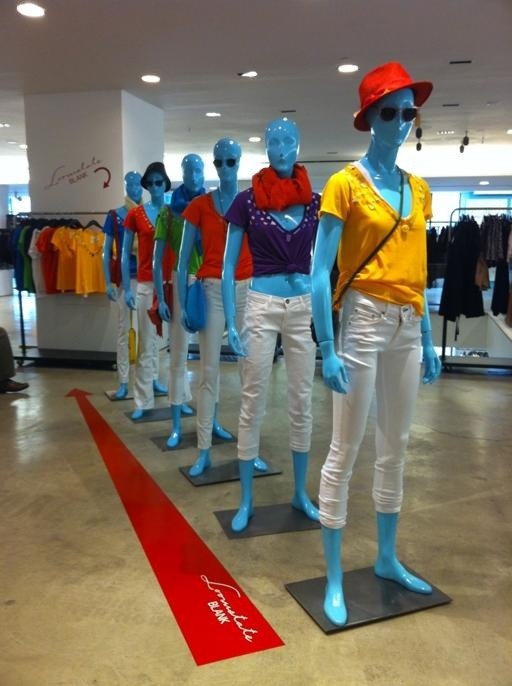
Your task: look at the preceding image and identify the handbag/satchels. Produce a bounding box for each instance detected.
[109,250,122,287]
[150,284,173,322]
[127,305,138,368]
[184,278,209,332]
[310,305,340,347]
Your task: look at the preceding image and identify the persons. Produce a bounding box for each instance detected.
[100,169,168,401]
[148,152,232,450]
[304,60,440,630]
[117,158,195,422]
[175,135,268,479]
[219,115,326,531]
[0,326,30,398]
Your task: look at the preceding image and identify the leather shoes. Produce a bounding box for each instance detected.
[0,377,29,394]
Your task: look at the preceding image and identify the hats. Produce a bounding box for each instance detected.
[354,60,434,132]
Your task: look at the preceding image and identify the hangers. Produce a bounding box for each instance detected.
[20,212,106,232]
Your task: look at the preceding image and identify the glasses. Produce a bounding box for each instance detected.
[370,106,418,122]
